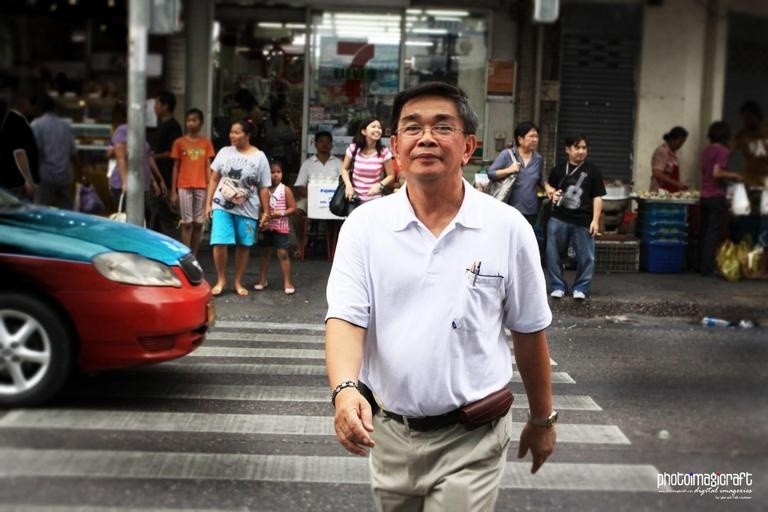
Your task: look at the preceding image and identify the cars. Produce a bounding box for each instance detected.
[1,185,213,406]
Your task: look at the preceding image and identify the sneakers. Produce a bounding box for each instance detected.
[282,281,297,297]
[550,288,567,299]
[572,288,586,301]
[253,279,269,292]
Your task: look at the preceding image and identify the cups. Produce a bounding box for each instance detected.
[554,192,566,207]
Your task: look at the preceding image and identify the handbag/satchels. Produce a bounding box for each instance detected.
[330,141,359,220]
[217,172,251,208]
[486,149,517,203]
[107,194,148,230]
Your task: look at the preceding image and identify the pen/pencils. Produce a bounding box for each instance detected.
[470,261,482,287]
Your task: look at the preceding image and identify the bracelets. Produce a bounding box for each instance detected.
[331,380,361,408]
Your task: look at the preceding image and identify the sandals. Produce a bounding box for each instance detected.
[233,283,250,298]
[210,280,227,297]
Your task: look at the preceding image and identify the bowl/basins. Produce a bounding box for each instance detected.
[600,193,630,214]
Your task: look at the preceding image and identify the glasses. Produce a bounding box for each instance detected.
[396,123,465,143]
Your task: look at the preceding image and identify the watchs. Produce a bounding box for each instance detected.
[527,410,559,428]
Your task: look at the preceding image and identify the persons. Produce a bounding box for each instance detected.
[0,66,767,302]
[323,80,558,512]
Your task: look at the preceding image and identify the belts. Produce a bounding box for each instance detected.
[381,409,462,435]
